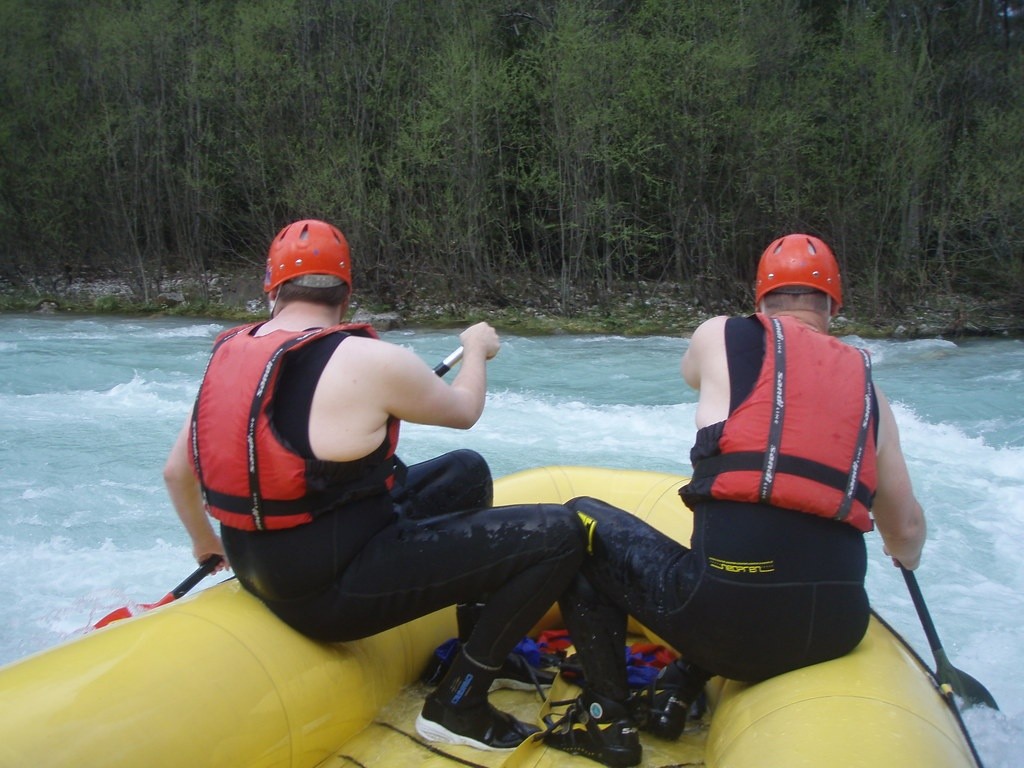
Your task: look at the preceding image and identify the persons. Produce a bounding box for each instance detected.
[543,234,927,768]
[163,220,587,752]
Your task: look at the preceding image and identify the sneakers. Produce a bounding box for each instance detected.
[487,649,559,702]
[415,690,542,752]
[619,657,704,741]
[541,695,643,767]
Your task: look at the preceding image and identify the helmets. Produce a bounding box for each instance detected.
[263,219,353,296]
[756,233,842,309]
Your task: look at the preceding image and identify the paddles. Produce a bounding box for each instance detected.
[89,344,468,633]
[898,561,1002,714]
